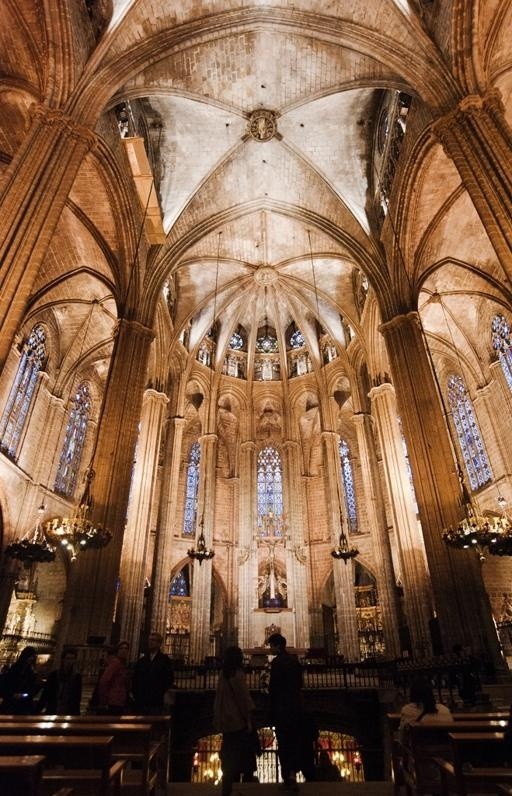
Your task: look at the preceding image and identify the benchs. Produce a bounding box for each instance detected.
[383,710,512,796]
[0,713,174,796]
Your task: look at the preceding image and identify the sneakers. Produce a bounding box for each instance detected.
[279,783,297,792]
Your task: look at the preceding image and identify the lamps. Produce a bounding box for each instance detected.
[182,231,225,564]
[40,118,161,565]
[356,117,512,564]
[308,228,361,565]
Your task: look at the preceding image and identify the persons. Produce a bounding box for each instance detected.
[0,632,175,769]
[393,641,511,796]
[211,633,320,796]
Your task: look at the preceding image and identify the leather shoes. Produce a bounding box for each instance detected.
[242,775,258,784]
